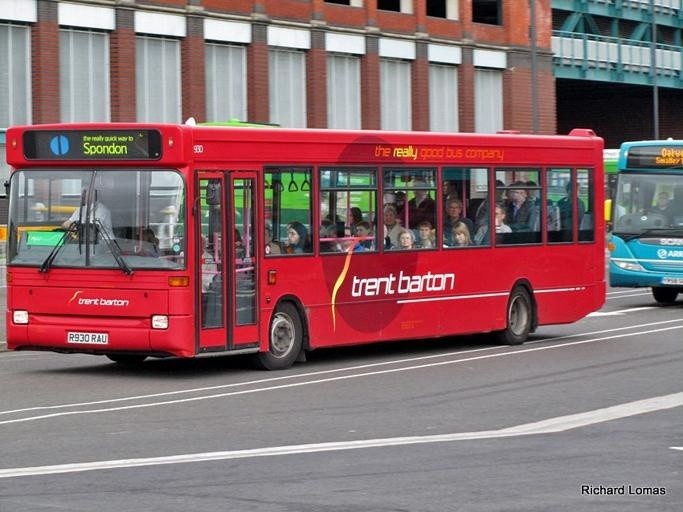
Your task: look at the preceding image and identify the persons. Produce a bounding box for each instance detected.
[647,191,671,227]
[60,185,115,244]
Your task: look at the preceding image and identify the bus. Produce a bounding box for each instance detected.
[527,136,682,305]
[4,115,613,373]
